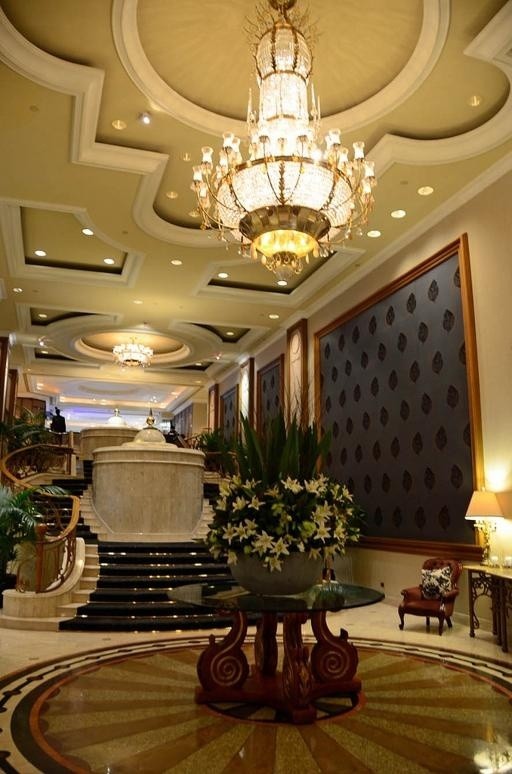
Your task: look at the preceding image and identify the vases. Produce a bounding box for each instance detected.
[230,553,323,595]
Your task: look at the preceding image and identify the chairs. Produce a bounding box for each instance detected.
[398,558,463,636]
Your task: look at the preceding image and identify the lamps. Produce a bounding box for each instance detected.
[112,338,153,368]
[191,0,376,285]
[464,488,504,568]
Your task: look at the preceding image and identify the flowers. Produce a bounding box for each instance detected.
[208,412,363,571]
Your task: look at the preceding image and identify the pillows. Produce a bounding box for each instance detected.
[421,567,451,599]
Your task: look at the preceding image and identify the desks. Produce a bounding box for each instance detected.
[169,580,386,726]
[463,566,512,652]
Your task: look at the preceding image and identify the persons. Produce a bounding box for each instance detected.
[167,425,179,443]
[51,408,66,446]
